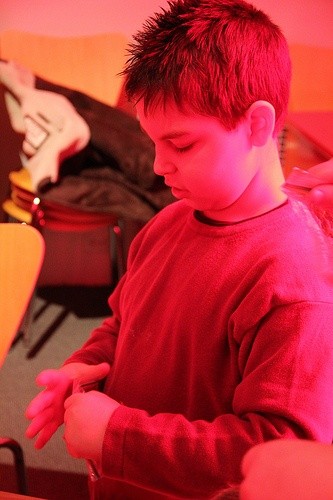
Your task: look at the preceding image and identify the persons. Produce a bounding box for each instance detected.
[24,1,333,498]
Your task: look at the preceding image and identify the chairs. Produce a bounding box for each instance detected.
[0,169,140,498]
